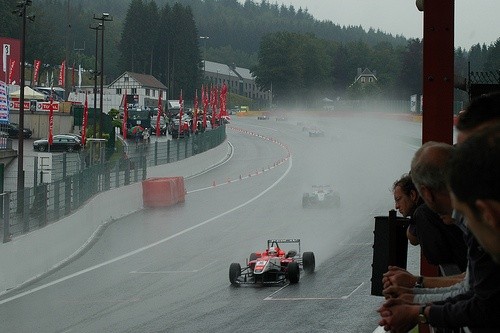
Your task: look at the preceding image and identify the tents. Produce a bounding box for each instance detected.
[8,86,44,102]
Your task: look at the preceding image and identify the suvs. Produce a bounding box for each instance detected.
[33,133,86,153]
[151,116,167,135]
[0,122,33,138]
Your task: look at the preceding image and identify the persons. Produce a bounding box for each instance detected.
[142,128,150,149]
[268,247,279,256]
[377,92,500,333]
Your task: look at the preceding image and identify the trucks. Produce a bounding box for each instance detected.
[241,105,249,112]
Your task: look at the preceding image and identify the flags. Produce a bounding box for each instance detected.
[58,61,64,85]
[48,82,227,145]
[33,59,41,81]
[9,61,16,83]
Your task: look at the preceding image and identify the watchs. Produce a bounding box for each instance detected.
[416,276,423,288]
[417,305,429,324]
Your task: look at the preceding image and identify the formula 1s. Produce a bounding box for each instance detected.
[228,238,315,285]
[301,184,340,208]
[257,115,324,137]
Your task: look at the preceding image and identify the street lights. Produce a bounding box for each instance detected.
[88,22,104,145]
[93,11,113,146]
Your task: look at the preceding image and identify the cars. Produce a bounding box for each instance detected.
[189,115,212,127]
[168,119,186,134]
